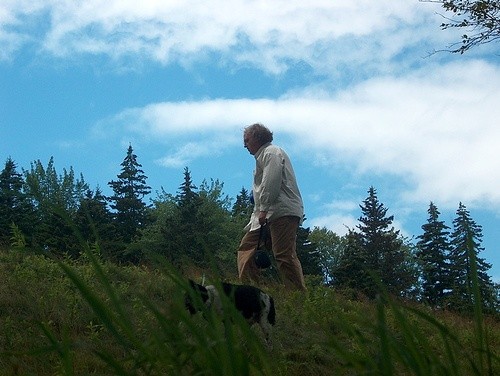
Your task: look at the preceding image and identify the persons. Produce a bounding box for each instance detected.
[235,123,308,302]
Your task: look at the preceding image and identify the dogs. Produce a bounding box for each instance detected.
[183,277,276,343]
